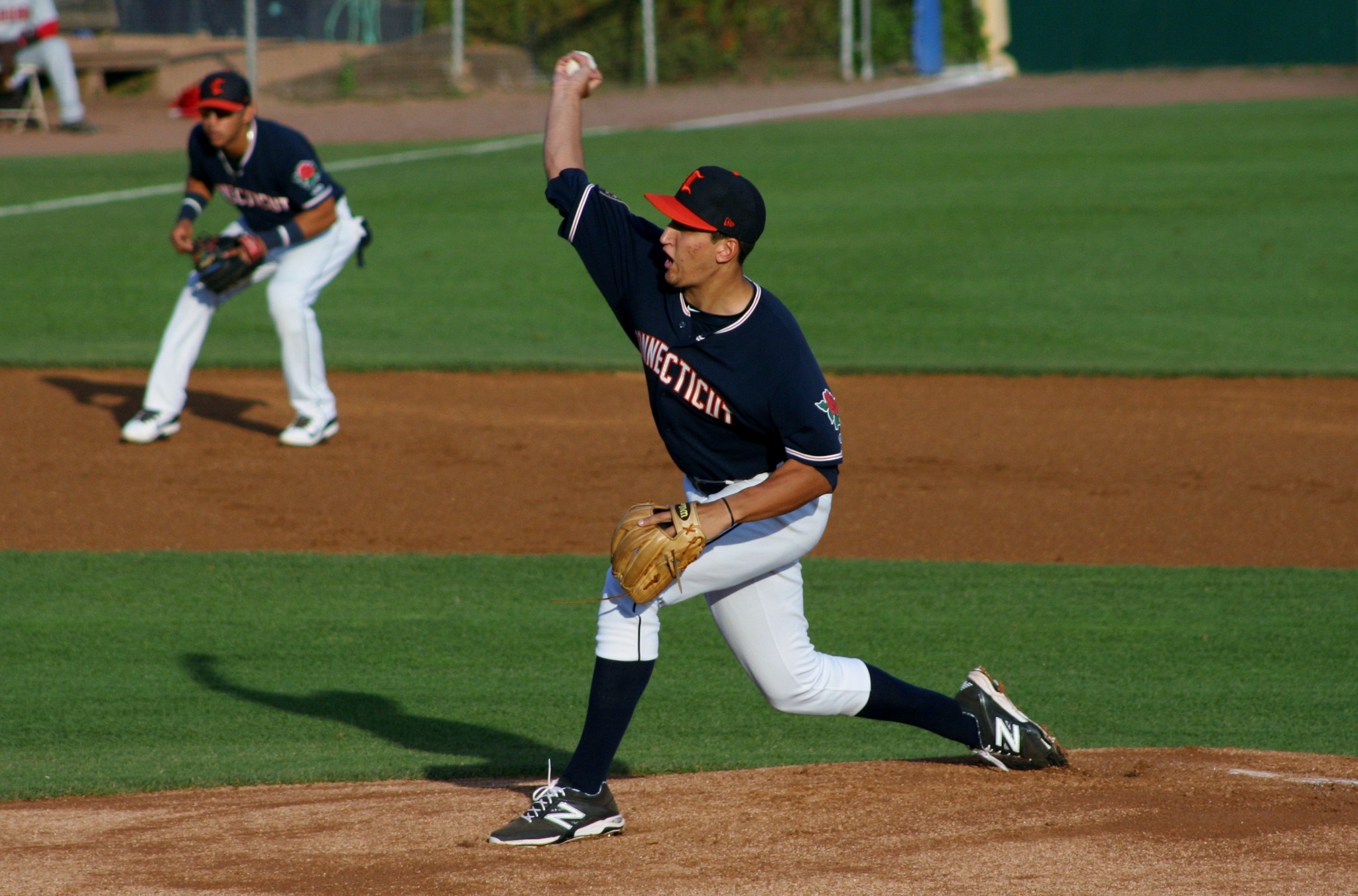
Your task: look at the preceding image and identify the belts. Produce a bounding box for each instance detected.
[685,474,736,497]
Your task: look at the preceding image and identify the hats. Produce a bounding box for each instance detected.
[197,70,253,112]
[642,165,769,240]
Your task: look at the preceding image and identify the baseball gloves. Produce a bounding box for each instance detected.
[610,499,706,603]
[192,232,264,292]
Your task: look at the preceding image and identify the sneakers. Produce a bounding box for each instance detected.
[119,405,182,446]
[279,410,340,448]
[483,757,626,844]
[959,667,1071,769]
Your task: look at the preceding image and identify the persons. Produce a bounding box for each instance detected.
[121,70,366,446]
[488,50,1067,845]
[0,0,87,132]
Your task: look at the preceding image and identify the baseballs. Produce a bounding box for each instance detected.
[564,50,597,76]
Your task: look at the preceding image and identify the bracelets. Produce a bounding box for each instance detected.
[721,497,734,526]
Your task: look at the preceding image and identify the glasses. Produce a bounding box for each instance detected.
[197,109,235,120]
